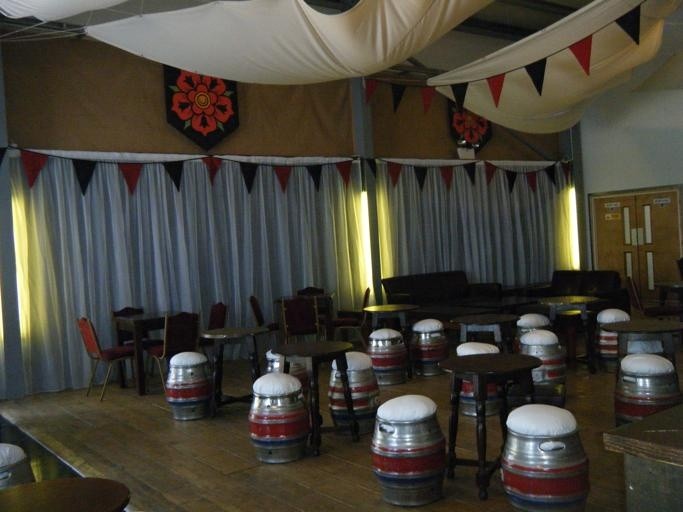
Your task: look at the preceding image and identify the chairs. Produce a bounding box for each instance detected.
[280,296,324,370]
[75,284,371,402]
[652,255,683,320]
[196,302,228,378]
[324,290,370,369]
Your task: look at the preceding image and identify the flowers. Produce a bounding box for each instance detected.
[168,68,236,139]
[451,105,492,152]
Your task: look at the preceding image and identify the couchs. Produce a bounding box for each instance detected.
[540,268,631,322]
[378,268,504,306]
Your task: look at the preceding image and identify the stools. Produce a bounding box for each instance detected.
[369,328,405,386]
[455,342,500,415]
[413,319,449,375]
[328,350,379,435]
[0,442,38,490]
[168,352,214,421]
[550,307,593,371]
[597,308,629,360]
[371,394,447,506]
[519,330,564,387]
[614,354,679,428]
[501,403,590,509]
[517,314,549,353]
[247,373,308,466]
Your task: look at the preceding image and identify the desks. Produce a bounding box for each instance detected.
[435,354,544,501]
[0,476,131,512]
[361,303,420,381]
[270,341,362,456]
[601,319,681,383]
[538,295,601,375]
[449,313,517,354]
[199,327,269,404]
